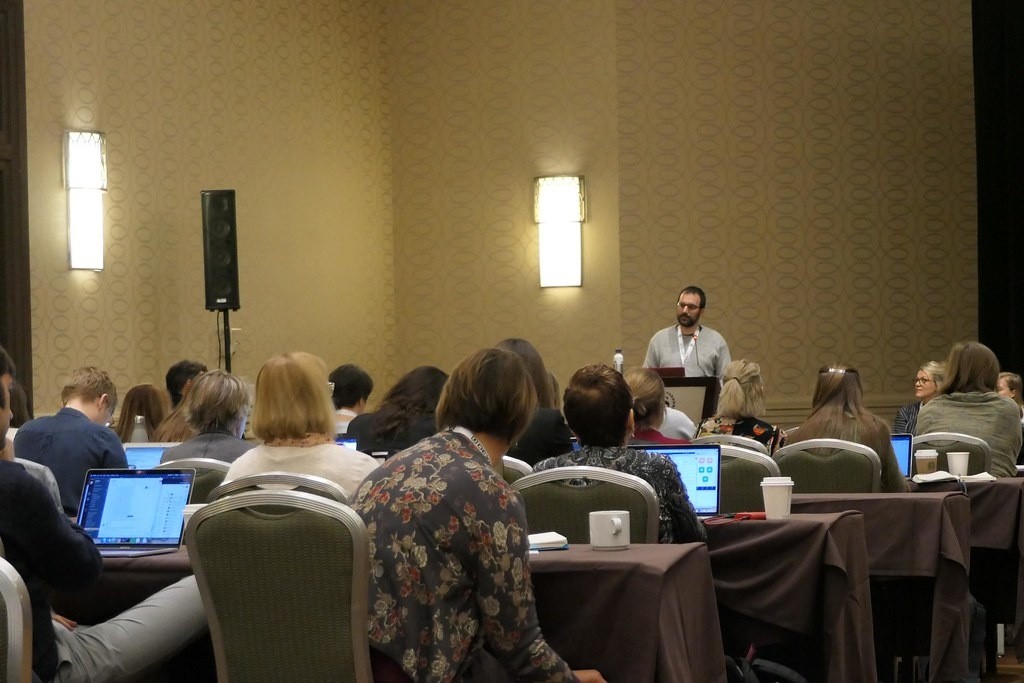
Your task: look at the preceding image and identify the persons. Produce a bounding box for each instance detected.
[14,366,129,524]
[623,368,692,444]
[328,363,374,445]
[160,368,258,505]
[534,363,708,544]
[642,286,731,389]
[348,366,449,461]
[783,360,911,493]
[693,360,788,457]
[226,351,379,498]
[347,348,606,683]
[890,342,1024,477]
[0,345,208,683]
[114,360,245,443]
[495,339,574,469]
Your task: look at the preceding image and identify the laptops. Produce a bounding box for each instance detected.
[75,442,196,557]
[890,433,913,481]
[626,443,721,521]
[645,367,684,377]
[334,431,359,451]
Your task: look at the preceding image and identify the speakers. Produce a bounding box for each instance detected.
[200,189,241,312]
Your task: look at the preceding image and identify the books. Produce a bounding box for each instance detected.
[527,531,570,551]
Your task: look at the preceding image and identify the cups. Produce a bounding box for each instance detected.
[589,511,630,551]
[182,502,208,529]
[914,450,939,474]
[946,452,970,476]
[760,477,795,520]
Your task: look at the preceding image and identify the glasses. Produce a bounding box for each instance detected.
[327,382,334,395]
[913,376,935,385]
[98,393,114,428]
[678,302,700,310]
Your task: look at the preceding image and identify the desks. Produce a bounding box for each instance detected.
[909,477,1024,671]
[101,543,727,683]
[792,493,985,683]
[704,512,878,683]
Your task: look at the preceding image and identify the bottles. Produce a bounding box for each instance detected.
[614,349,623,375]
[132,416,148,442]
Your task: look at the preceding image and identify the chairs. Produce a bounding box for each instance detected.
[502,457,660,543]
[689,436,881,512]
[913,432,992,476]
[154,456,374,683]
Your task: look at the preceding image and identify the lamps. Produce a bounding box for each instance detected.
[534,174,586,288]
[61,127,107,270]
